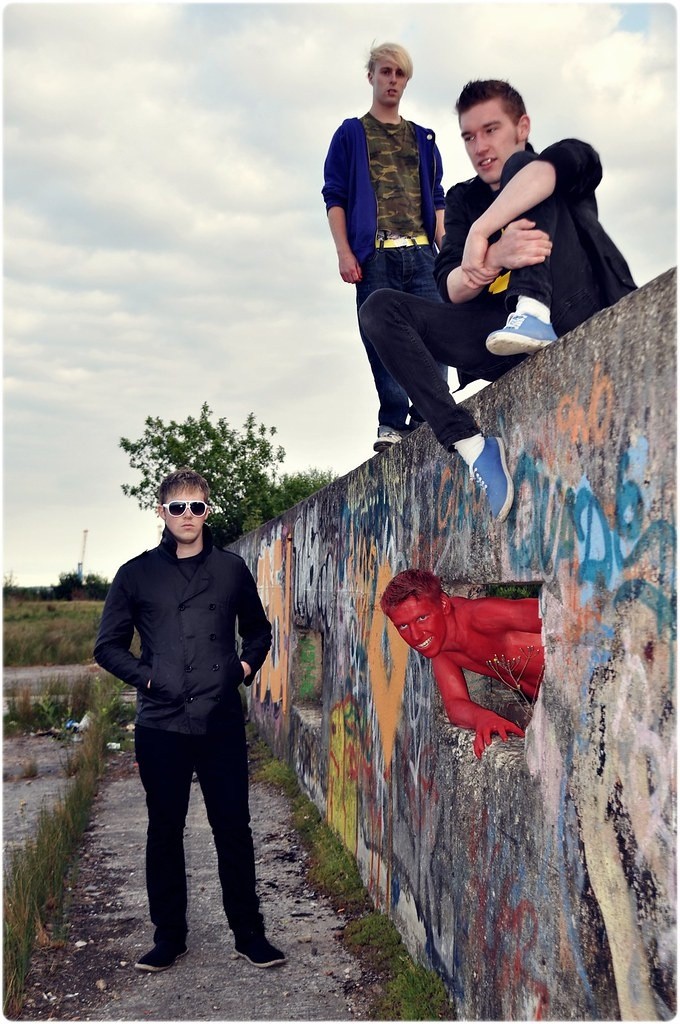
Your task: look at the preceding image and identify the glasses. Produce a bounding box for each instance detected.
[162,501,211,518]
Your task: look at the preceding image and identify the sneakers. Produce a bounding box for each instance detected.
[134,944,189,971]
[486,311,557,356]
[408,419,422,430]
[373,425,402,452]
[468,437,514,523]
[233,936,286,967]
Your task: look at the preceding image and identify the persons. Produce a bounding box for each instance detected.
[357,79,638,525]
[91,471,287,972]
[378,568,544,762]
[321,44,447,454]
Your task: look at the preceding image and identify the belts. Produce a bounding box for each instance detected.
[376,236,429,248]
